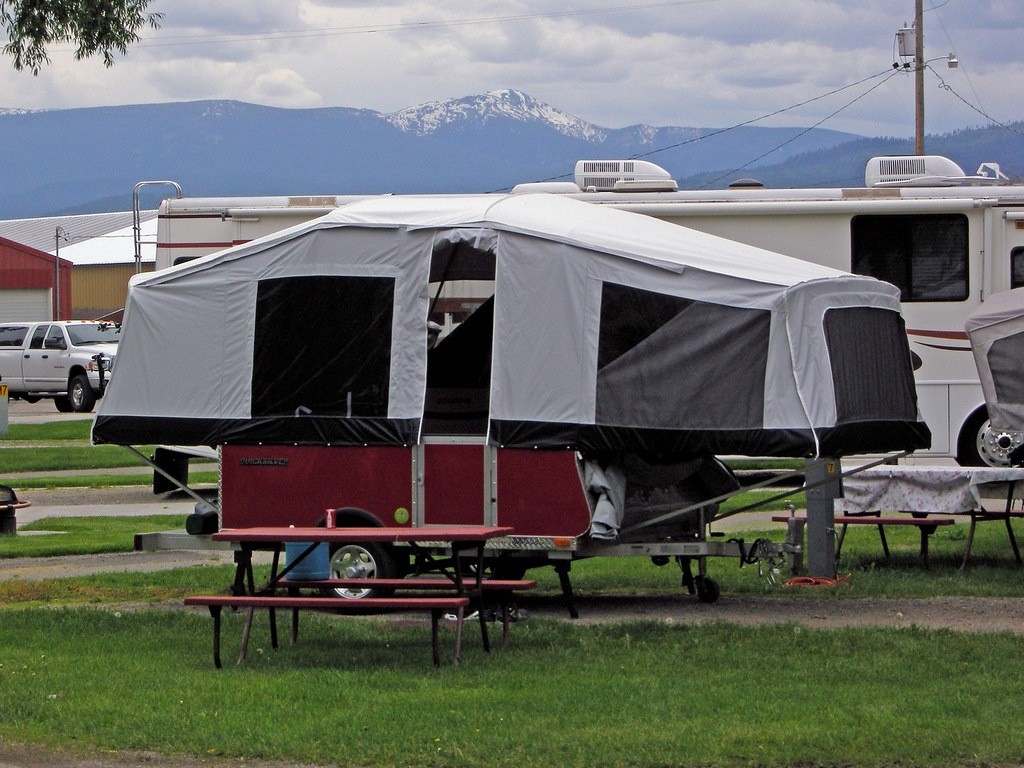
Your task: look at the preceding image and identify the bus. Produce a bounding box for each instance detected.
[131,154,1023,486]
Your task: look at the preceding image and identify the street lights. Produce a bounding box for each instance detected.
[54,226,68,320]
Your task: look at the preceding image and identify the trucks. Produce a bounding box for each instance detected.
[0,318,123,414]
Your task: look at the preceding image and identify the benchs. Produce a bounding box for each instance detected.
[182,595,472,673]
[906,509,1024,568]
[771,511,954,575]
[254,575,536,659]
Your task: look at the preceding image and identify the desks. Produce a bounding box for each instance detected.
[832,464,1024,574]
[210,523,513,671]
